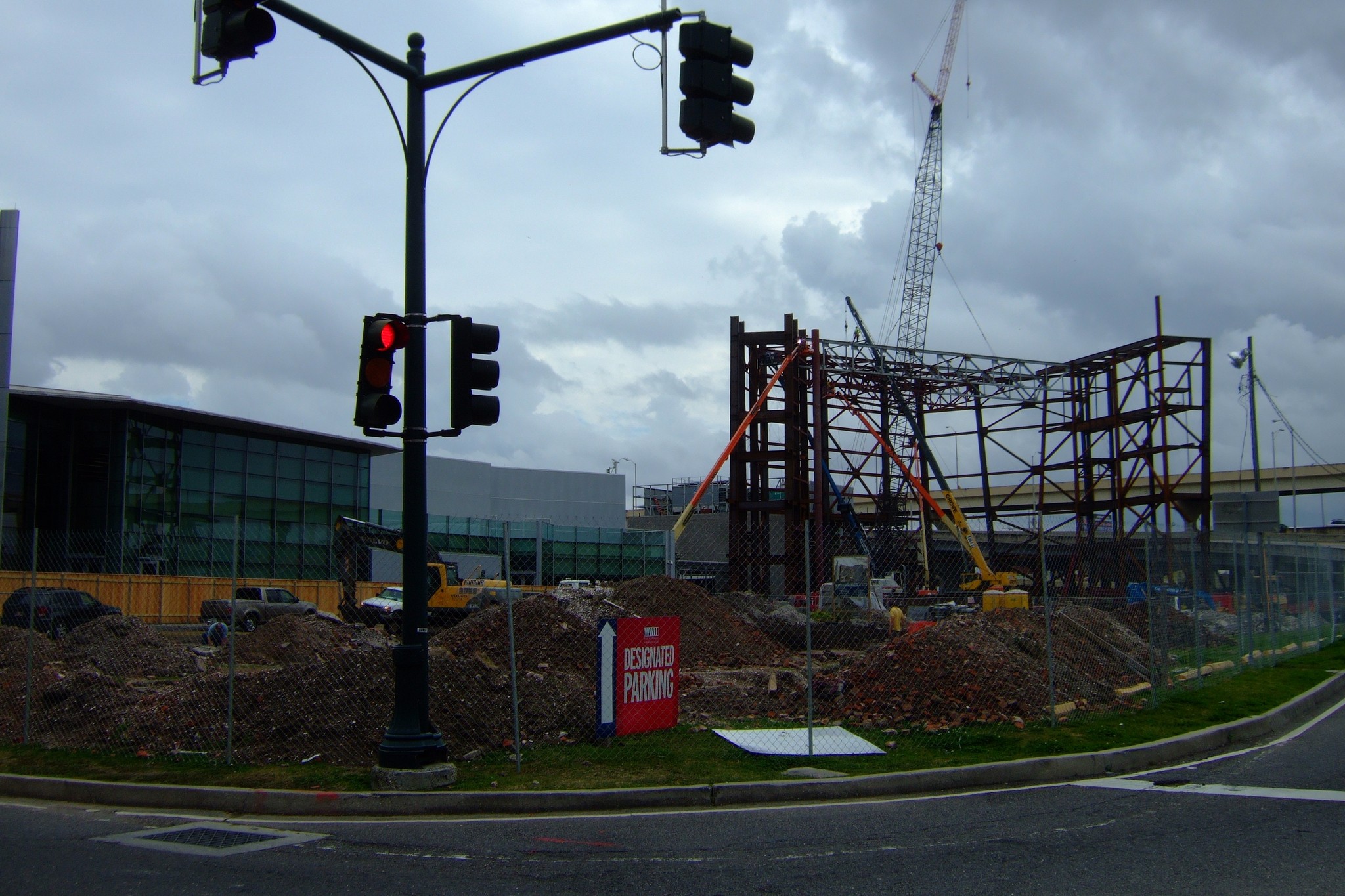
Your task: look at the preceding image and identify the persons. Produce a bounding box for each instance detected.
[200,622,228,647]
[889,601,905,639]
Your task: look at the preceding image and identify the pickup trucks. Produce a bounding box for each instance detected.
[200,586,317,633]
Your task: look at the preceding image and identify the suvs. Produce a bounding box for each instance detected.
[4,588,122,640]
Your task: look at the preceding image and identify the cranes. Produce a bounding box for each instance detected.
[870,1,971,550]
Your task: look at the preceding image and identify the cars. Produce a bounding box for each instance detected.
[1326,519,1345,527]
[360,586,402,618]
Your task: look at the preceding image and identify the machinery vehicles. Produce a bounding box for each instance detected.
[1122,575,1288,611]
[758,351,905,607]
[821,296,1017,592]
[333,516,590,629]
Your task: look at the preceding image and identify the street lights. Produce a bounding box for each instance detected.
[1272,419,1297,533]
[1176,402,1190,474]
[615,458,637,509]
[1272,429,1284,492]
[1227,336,1271,634]
[1032,451,1041,530]
[946,426,959,490]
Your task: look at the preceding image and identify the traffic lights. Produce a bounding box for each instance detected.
[679,21,754,149]
[203,0,276,60]
[451,317,500,426]
[354,316,409,428]
